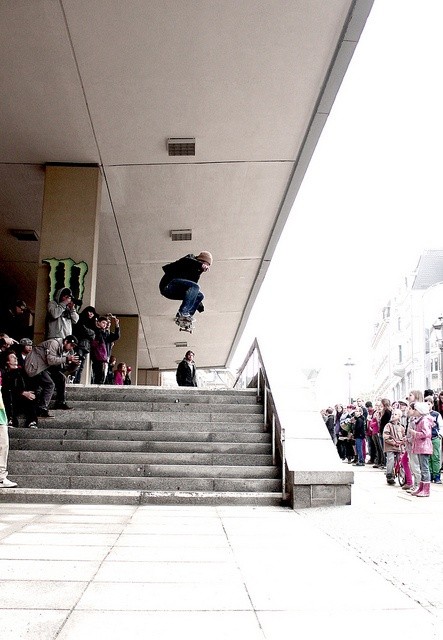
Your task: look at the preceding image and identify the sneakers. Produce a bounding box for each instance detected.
[28,420,39,429]
[405,486,415,493]
[54,401,75,410]
[386,473,394,484]
[1,478,18,487]
[8,418,19,428]
[179,311,190,317]
[175,313,196,321]
[40,408,55,419]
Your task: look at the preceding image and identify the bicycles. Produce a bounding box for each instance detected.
[389,436,408,486]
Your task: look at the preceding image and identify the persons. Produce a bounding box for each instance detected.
[320,398,378,466]
[377,390,443,497]
[124,366,132,385]
[18,338,32,366]
[5,300,26,338]
[0,384,17,488]
[105,356,116,384]
[26,335,81,419]
[0,334,33,366]
[114,362,127,385]
[72,306,96,384]
[176,350,198,386]
[159,251,212,322]
[103,313,121,384]
[1,332,15,369]
[92,316,110,384]
[44,287,80,338]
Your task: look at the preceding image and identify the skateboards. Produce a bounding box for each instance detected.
[175,316,193,334]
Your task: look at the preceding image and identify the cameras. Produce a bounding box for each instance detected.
[74,354,85,362]
[68,295,83,306]
[111,316,116,325]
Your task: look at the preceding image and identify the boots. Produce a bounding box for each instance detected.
[429,473,432,482]
[411,481,423,496]
[434,474,441,484]
[416,482,430,497]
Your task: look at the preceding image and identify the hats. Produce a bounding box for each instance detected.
[20,338,34,346]
[414,402,429,415]
[197,251,212,265]
[67,335,78,346]
